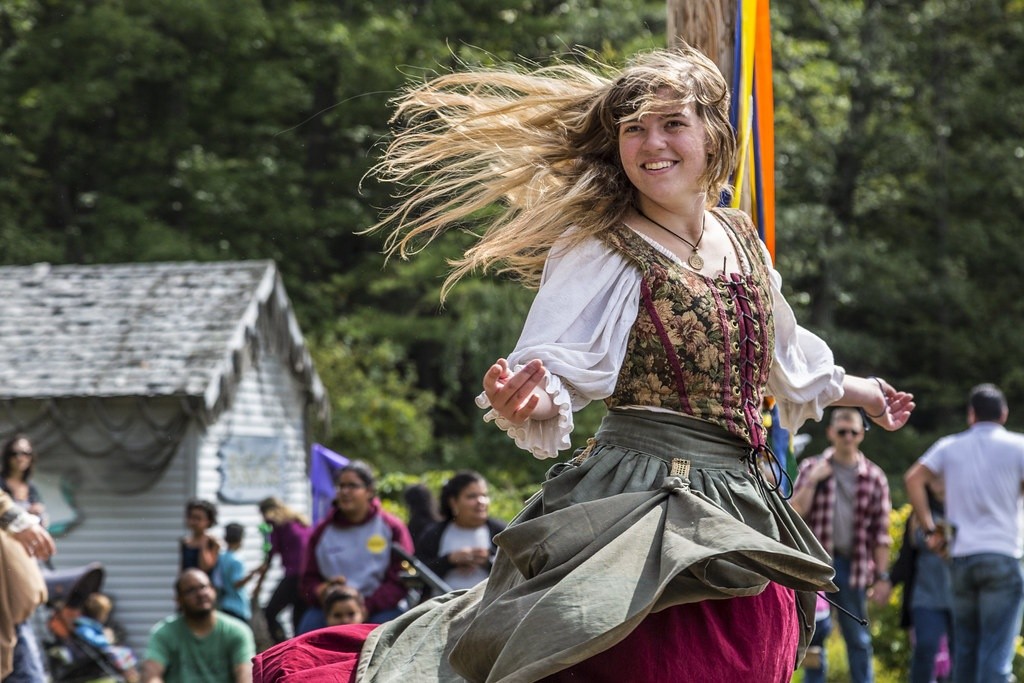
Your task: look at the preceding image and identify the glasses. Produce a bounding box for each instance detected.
[9,450,33,458]
[339,481,365,490]
[832,427,861,438]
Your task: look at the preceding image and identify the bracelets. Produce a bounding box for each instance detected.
[925,528,938,535]
[864,376,888,418]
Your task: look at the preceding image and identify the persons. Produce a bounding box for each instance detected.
[405,487,437,542]
[325,588,367,627]
[252,50,916,683]
[790,409,890,683]
[177,500,265,621]
[144,569,257,683]
[253,498,309,644]
[905,388,1024,683]
[0,436,56,683]
[418,471,507,605]
[889,508,954,683]
[296,465,412,636]
[71,593,140,683]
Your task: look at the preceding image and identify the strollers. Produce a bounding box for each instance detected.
[8,557,141,683]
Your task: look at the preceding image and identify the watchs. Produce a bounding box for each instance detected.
[875,572,888,580]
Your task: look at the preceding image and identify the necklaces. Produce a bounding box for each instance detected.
[633,203,706,270]
